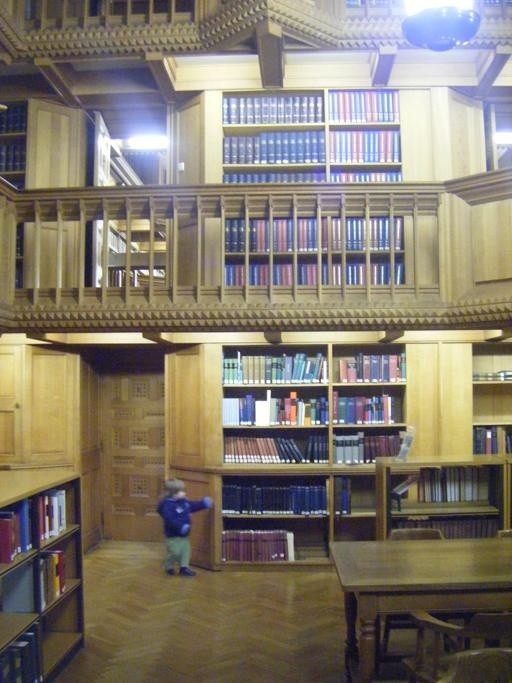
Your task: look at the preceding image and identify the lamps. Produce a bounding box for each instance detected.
[398,0,480,52]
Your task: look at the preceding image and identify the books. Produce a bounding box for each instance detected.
[0,487,68,683]
[0,103,27,186]
[223,477,351,514]
[472,371,512,381]
[390,465,500,538]
[225,432,400,464]
[474,425,512,455]
[224,389,395,426]
[225,349,406,384]
[223,90,406,285]
[224,529,294,559]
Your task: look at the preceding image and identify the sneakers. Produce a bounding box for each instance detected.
[167,566,196,577]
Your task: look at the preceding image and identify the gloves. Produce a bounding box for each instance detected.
[204,497,212,509]
[181,524,190,535]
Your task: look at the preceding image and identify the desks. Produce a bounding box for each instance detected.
[328,537,512,683]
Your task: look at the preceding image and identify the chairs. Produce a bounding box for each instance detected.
[372,529,512,683]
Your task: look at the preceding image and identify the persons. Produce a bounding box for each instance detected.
[158,477,215,576]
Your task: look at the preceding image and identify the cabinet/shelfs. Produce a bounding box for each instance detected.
[220,87,404,183]
[0,478,86,683]
